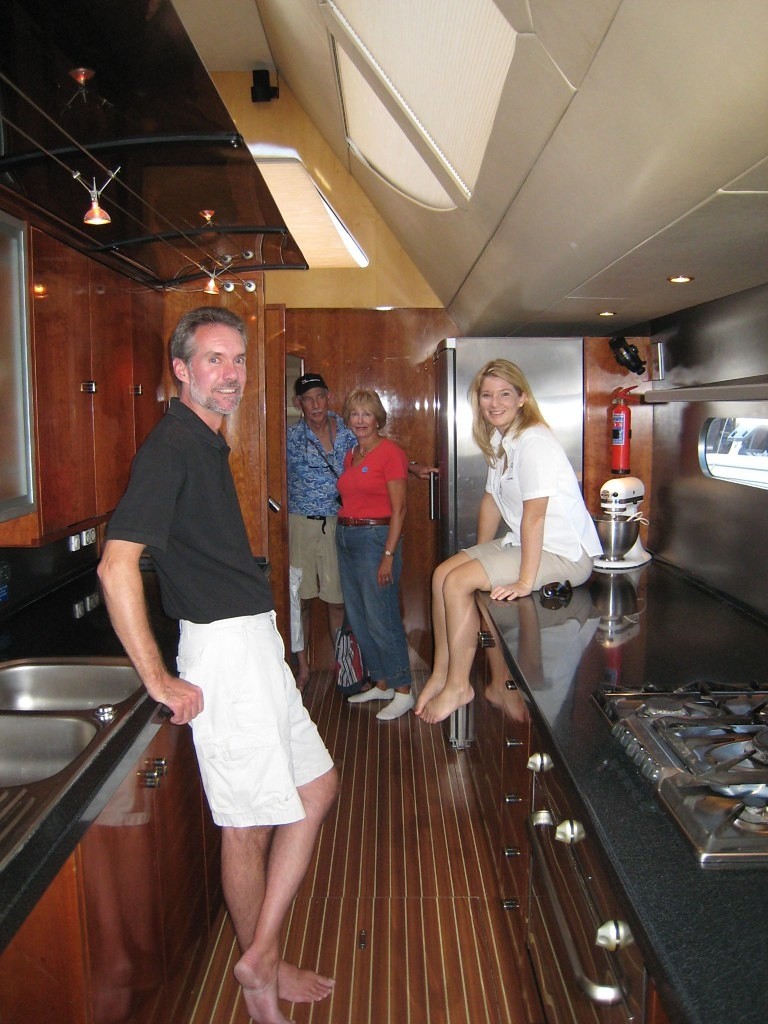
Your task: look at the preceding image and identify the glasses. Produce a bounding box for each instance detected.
[301,396,327,401]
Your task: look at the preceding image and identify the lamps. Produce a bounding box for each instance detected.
[59,68,113,119]
[200,211,218,227]
[201,265,233,295]
[68,165,121,225]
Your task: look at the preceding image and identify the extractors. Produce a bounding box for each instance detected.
[645,374,768,403]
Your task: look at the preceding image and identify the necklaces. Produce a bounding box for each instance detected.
[360,435,380,457]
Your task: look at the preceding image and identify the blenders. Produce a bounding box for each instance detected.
[593,477,652,568]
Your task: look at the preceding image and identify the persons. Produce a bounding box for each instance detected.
[96,306,338,1024]
[414,357,604,724]
[337,387,414,719]
[287,373,438,691]
[476,586,601,727]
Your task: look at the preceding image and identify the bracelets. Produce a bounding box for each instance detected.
[409,461,417,464]
[384,550,394,557]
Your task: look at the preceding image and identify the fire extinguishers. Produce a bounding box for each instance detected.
[610,385,639,475]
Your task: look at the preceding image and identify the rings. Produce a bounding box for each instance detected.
[385,578,390,580]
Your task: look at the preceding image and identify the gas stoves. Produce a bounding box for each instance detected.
[591,681,768,862]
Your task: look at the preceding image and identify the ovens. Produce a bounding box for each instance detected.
[520,730,647,1024]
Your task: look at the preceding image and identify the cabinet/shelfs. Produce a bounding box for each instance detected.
[0,210,167,548]
[0,710,223,1024]
[468,603,671,1024]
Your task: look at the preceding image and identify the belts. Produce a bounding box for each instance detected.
[336,516,391,527]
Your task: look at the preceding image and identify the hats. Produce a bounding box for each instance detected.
[295,374,328,396]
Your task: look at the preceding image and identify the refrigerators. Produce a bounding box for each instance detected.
[429,336,586,750]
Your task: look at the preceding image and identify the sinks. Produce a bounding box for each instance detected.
[0,715,97,787]
[0,664,143,710]
